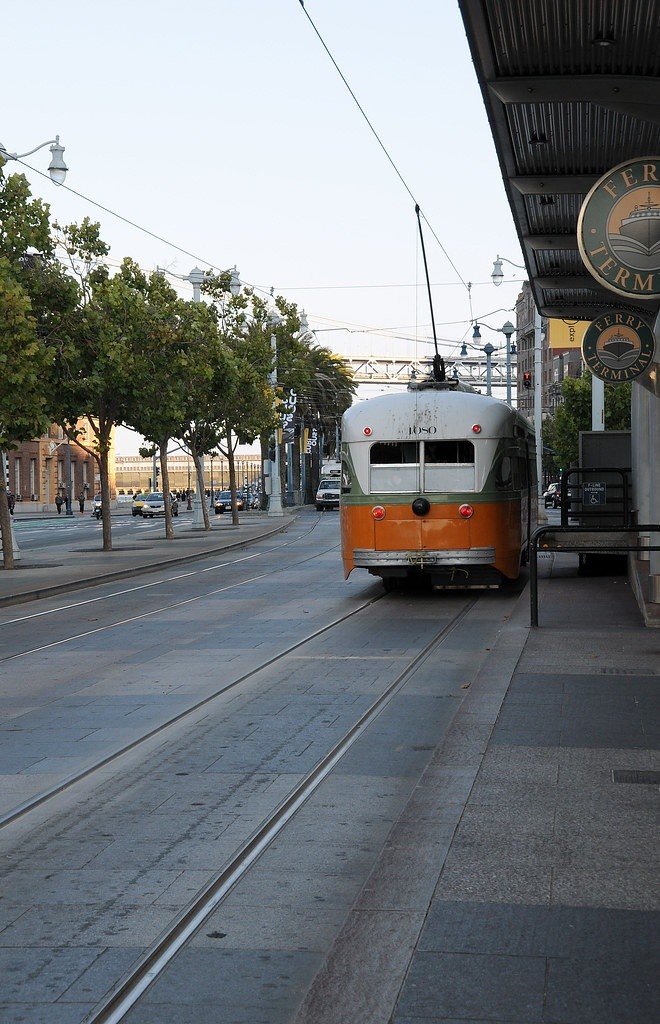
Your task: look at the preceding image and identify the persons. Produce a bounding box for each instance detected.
[55,493,68,514]
[176,488,190,501]
[215,486,230,498]
[7,491,16,515]
[133,493,140,501]
[78,492,85,513]
[205,488,210,497]
[92,491,102,519]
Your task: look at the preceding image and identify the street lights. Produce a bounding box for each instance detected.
[460,341,517,396]
[472,318,546,404]
[490,255,548,524]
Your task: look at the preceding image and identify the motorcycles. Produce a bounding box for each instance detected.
[92,491,102,520]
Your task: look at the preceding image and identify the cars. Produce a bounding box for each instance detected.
[314,477,342,511]
[215,490,243,514]
[132,493,150,517]
[141,492,178,518]
[543,483,571,509]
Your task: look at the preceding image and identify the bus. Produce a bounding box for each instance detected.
[341,377,543,593]
